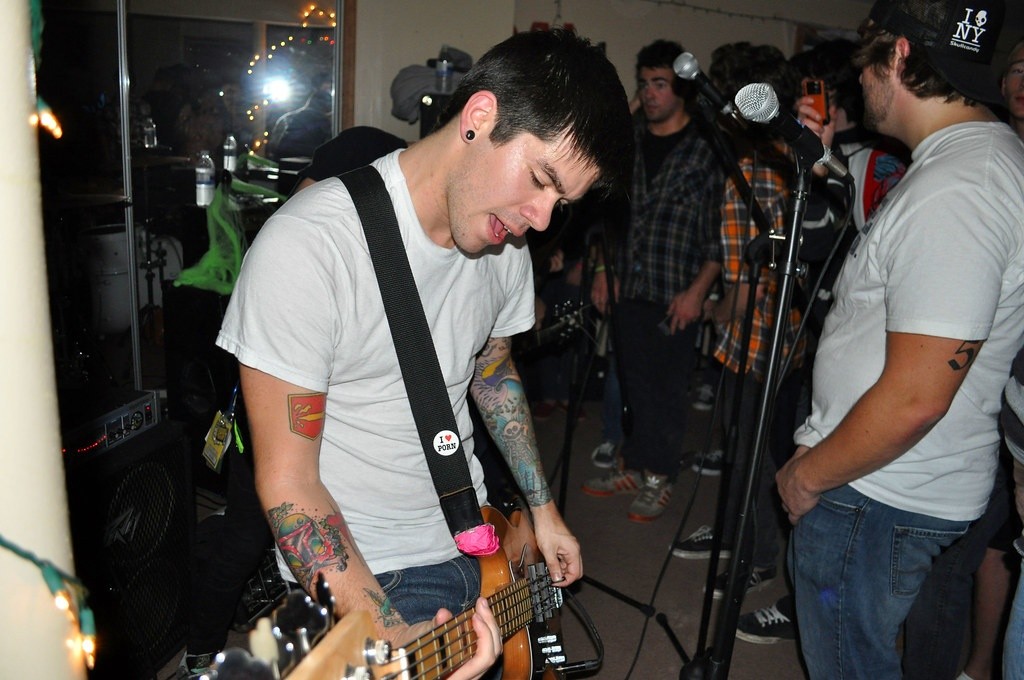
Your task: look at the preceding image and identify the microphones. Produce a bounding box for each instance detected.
[734,83,854,183]
[674,52,748,131]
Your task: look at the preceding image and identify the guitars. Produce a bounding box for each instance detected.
[197,567,396,680]
[340,501,567,679]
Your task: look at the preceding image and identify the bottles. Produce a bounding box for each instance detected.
[144,117,157,148]
[194,149,215,207]
[222,134,238,172]
[435,44,454,94]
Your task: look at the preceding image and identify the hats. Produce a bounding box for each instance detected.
[868,0,998,99]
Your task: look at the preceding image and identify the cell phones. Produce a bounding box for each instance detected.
[801,80,830,125]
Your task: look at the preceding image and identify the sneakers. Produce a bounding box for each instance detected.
[666,524,733,559]
[703,561,778,600]
[692,382,714,411]
[692,448,723,476]
[590,441,617,469]
[584,454,649,496]
[735,596,803,645]
[626,467,681,522]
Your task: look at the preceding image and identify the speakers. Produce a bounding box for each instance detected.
[51,418,193,679]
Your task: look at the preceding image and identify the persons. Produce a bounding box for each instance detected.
[750,0,1024,680]
[215,34,631,680]
[106,67,332,196]
[290,40,1024,680]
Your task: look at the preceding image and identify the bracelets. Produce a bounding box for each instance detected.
[594,264,614,273]
[575,263,582,269]
